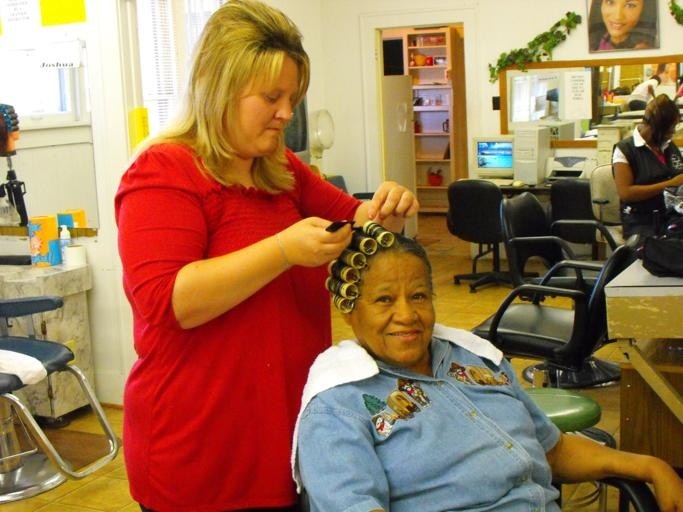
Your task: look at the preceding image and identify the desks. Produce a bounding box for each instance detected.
[0,263,96,418]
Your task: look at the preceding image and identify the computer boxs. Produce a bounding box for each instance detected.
[513,126,551,185]
[539,122,575,140]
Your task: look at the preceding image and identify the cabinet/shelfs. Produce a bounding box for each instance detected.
[402,29,456,214]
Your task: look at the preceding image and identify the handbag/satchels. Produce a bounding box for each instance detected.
[642,217,682,278]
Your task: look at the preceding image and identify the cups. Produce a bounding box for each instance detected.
[408,35,449,187]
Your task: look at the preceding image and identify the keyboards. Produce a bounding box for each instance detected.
[457,178,513,185]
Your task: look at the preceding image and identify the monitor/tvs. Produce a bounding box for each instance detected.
[473,135,513,178]
[283,95,310,164]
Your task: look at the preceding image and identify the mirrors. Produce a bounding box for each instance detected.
[500,53,683,150]
[0,0,100,229]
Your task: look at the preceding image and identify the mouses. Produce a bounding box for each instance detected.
[512,180,525,186]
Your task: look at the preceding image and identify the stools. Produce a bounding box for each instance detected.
[521,387,607,512]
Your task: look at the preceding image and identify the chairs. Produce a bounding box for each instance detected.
[545,180,606,256]
[592,164,623,244]
[0,297,119,503]
[469,233,642,450]
[499,191,621,390]
[447,179,540,294]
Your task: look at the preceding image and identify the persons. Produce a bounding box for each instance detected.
[112,1,420,511]
[625,62,683,112]
[289,217,682,511]
[610,92,683,244]
[586,0,656,53]
